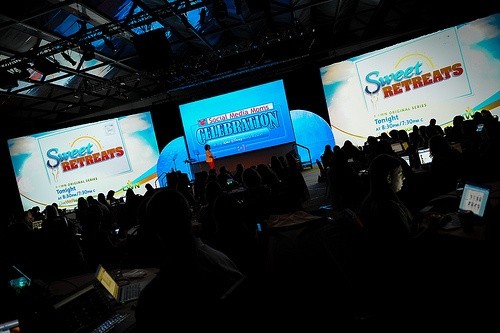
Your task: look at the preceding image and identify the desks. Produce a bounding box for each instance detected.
[411,181,500,245]
[46,269,160,333]
[266,211,328,239]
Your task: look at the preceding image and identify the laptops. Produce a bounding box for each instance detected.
[416,147,433,165]
[94,264,147,305]
[50,280,137,333]
[392,142,409,153]
[401,155,411,167]
[439,182,489,231]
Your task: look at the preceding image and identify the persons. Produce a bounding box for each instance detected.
[7,183,140,333]
[203,194,298,333]
[323,109,500,186]
[134,189,247,333]
[299,209,416,333]
[204,144,215,170]
[362,155,445,261]
[143,152,310,234]
[457,189,500,319]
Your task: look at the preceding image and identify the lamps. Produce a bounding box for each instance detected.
[34,56,58,75]
[103,34,121,56]
[80,43,95,61]
